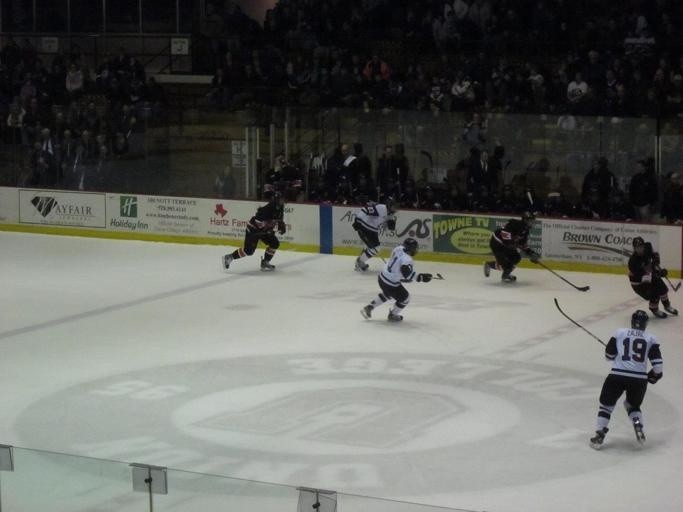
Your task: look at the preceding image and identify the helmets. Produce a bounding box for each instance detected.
[387,197,399,213]
[632,237,644,254]
[631,309,648,330]
[271,191,284,211]
[523,211,536,227]
[404,239,417,256]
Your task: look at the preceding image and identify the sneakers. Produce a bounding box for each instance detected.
[635,417,644,439]
[654,310,666,318]
[665,305,678,315]
[388,308,403,321]
[225,254,233,268]
[590,427,608,445]
[354,261,369,272]
[261,257,275,269]
[364,305,374,318]
[502,273,516,281]
[484,261,490,276]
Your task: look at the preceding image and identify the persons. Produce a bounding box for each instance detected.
[483,211,540,279]
[589,310,664,445]
[225,195,286,269]
[352,197,395,270]
[363,238,432,320]
[0,36,165,187]
[627,235,677,319]
[189,1,682,224]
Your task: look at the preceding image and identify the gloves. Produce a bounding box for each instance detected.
[420,274,432,282]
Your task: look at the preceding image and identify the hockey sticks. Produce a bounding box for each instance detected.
[247,225,281,235]
[426,273,444,280]
[422,150,434,183]
[554,298,606,347]
[536,261,589,291]
[657,264,682,292]
